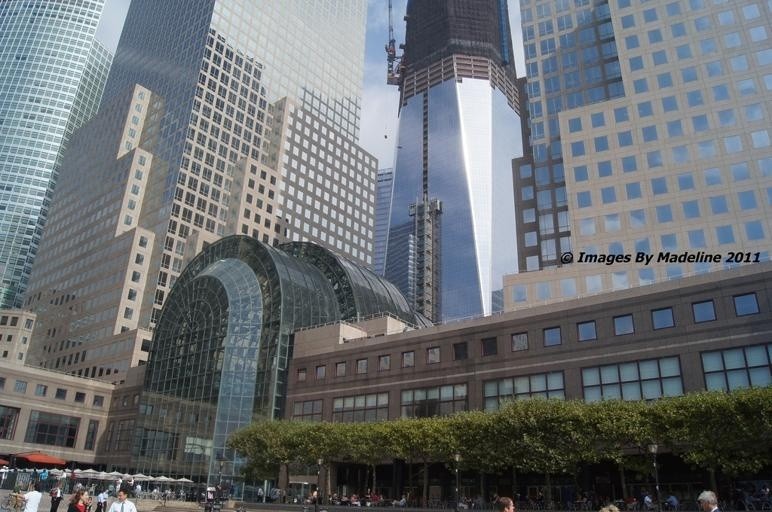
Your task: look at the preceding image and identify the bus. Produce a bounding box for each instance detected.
[289,481,311,499]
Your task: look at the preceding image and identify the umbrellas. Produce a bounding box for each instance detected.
[13,451,66,468]
[0,459,10,465]
[0,464,195,495]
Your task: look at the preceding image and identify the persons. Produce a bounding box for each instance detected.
[235,503,247,512]
[255,478,772,511]
[5,468,236,511]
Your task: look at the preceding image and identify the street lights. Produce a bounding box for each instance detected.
[219,460,224,484]
[451,450,462,512]
[316,456,324,505]
[646,443,664,512]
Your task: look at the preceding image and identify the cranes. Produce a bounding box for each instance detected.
[385,0,402,85]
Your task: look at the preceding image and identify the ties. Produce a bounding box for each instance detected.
[119,502,125,512]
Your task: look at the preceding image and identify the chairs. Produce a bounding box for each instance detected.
[515,500,700,512]
[307,496,478,508]
[133,487,216,503]
